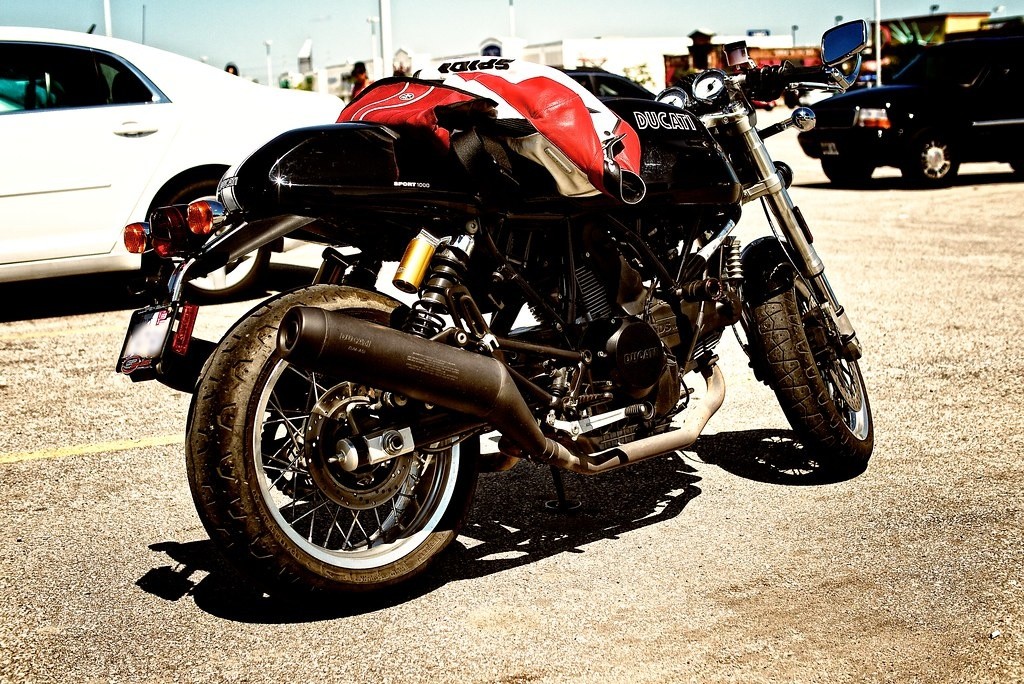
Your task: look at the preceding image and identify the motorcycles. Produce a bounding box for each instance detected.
[116,19,874,605]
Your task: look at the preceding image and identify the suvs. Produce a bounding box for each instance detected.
[795,30,1024,189]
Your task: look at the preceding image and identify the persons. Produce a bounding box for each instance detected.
[224,64,239,76]
[296,76,314,90]
[350,62,375,100]
[394,50,413,76]
[481,37,503,56]
[280,80,290,89]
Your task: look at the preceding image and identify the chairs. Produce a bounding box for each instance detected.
[73,52,111,105]
[40,79,65,108]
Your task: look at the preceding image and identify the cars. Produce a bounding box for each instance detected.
[0,27,346,303]
[558,67,656,100]
